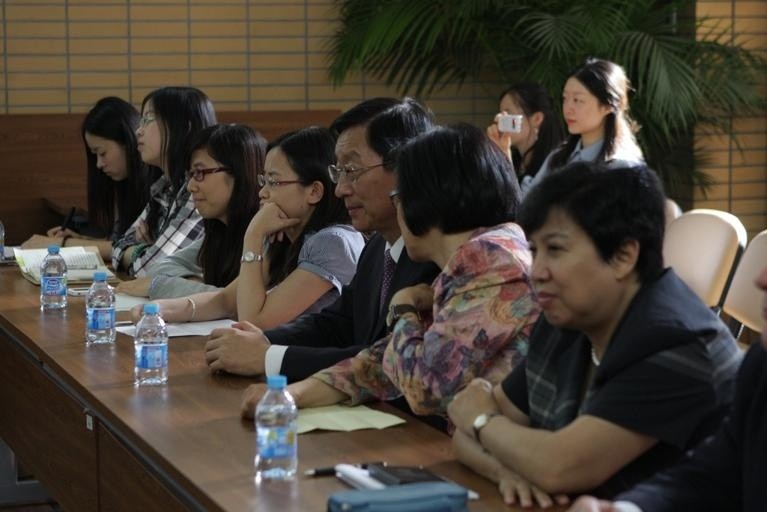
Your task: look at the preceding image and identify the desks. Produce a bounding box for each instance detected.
[0,246,563,512]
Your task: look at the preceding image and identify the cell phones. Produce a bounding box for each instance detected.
[68,287,90,296]
[370,464,445,488]
[497,114,523,133]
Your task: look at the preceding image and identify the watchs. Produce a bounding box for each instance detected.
[384,303,423,333]
[471,407,502,443]
[240,250,263,264]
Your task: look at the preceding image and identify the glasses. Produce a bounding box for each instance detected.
[139,116,156,127]
[388,190,398,204]
[257,171,299,188]
[328,163,383,183]
[185,165,228,181]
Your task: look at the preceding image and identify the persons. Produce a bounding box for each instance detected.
[202,95,441,384]
[108,86,216,280]
[563,267,767,511]
[129,124,371,332]
[111,122,270,303]
[239,120,541,439]
[444,158,745,510]
[484,58,643,197]
[18,96,163,262]
[495,81,562,185]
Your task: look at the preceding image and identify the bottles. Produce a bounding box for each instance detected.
[85,270,115,346]
[253,372,299,479]
[40,243,68,310]
[132,303,169,385]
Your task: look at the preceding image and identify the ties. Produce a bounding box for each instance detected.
[379,248,396,310]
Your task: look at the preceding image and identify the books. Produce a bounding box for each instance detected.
[0,246,16,263]
[7,262,17,266]
[12,244,122,285]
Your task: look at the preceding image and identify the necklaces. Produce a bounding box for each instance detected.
[588,346,600,367]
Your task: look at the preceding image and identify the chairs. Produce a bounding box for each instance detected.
[661,204,742,309]
[724,222,765,358]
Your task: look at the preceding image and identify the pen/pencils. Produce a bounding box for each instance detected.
[304,459,388,479]
[61,206,77,231]
[65,262,101,272]
[115,321,134,327]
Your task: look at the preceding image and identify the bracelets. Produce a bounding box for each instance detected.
[183,296,196,323]
[61,234,73,248]
